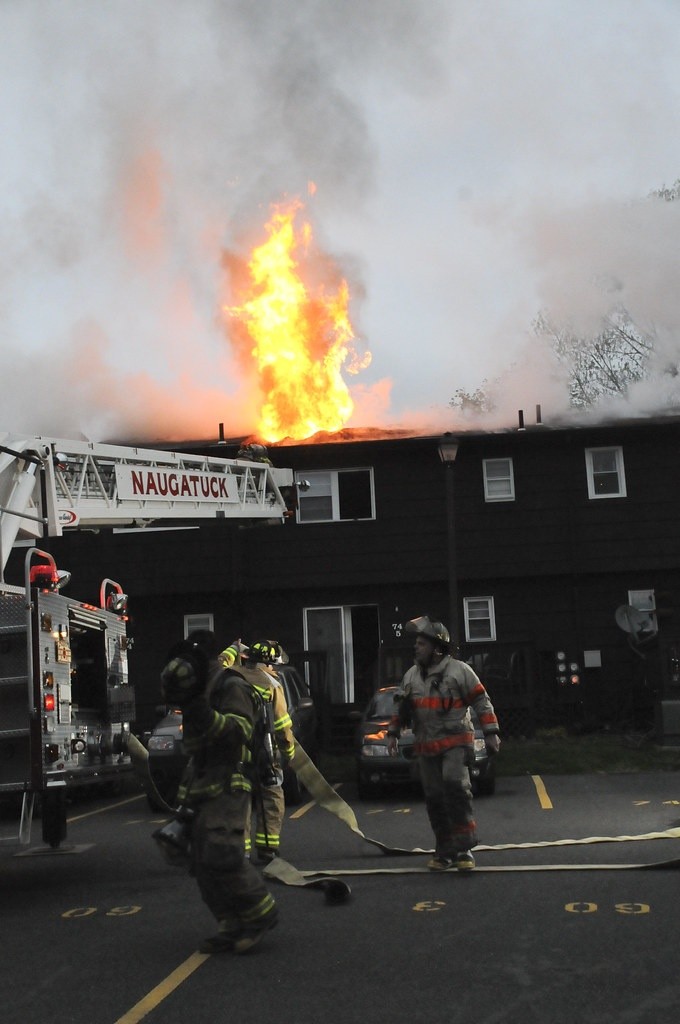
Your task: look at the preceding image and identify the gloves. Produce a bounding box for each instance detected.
[181,701,215,731]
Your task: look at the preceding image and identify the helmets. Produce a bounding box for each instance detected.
[418,621,454,651]
[160,657,199,709]
[245,640,280,664]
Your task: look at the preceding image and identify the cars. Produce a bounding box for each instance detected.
[348,685,494,802]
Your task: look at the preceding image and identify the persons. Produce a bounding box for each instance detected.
[387,617,500,871]
[160,628,297,956]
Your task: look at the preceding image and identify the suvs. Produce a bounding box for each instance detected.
[144,666,318,809]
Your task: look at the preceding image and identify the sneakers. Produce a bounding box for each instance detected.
[456,848,475,869]
[234,914,282,952]
[426,854,457,868]
[217,913,237,932]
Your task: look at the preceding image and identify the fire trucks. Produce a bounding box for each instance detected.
[0,434,294,818]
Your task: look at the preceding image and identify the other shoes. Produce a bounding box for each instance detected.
[255,847,279,863]
